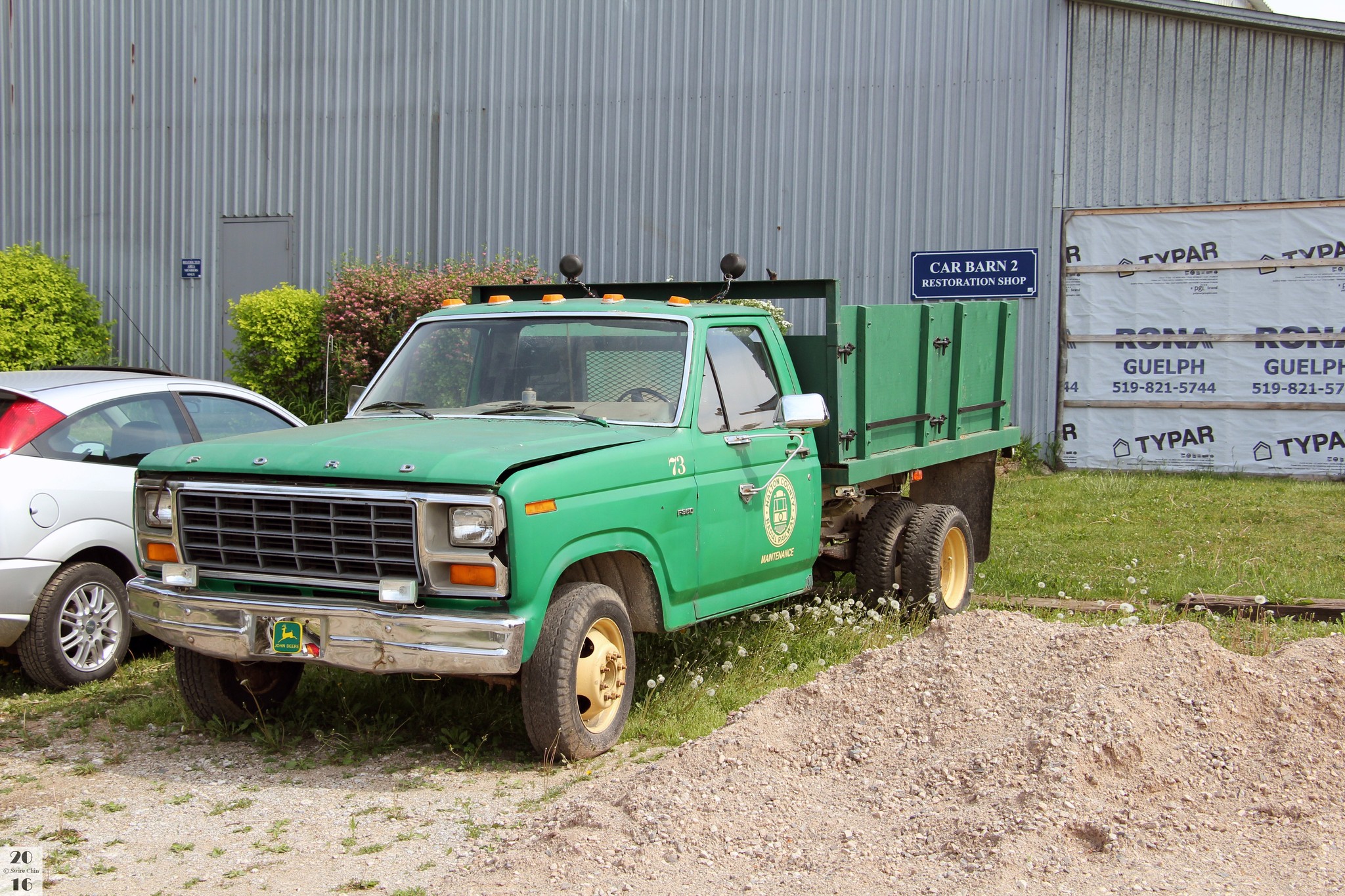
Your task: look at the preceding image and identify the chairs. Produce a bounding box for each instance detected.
[111,421,168,459]
[49,424,75,452]
[508,349,570,403]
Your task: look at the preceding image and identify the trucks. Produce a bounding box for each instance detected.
[125,252,1022,756]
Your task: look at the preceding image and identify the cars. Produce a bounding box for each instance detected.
[0,362,308,695]
